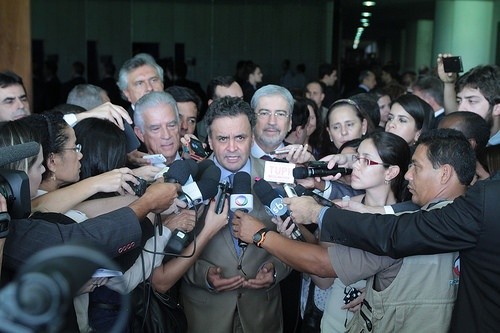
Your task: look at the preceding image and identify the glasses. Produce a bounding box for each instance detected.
[62,143,83,154]
[351,155,384,168]
[257,111,290,119]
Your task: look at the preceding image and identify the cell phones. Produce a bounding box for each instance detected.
[442,56,464,73]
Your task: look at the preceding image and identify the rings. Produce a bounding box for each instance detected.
[289,212,293,217]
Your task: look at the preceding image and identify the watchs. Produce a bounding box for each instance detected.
[252,226,273,249]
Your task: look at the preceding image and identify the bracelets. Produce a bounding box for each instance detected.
[273,272,276,279]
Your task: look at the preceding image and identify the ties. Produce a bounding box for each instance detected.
[227,174,242,258]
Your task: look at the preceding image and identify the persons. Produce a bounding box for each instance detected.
[283,172,500,333]
[0,52,500,333]
[178,96,304,332]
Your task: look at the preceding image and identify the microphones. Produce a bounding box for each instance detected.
[1,141,40,166]
[231,171,252,250]
[165,154,222,253]
[270,148,304,154]
[253,180,304,242]
[293,166,354,179]
